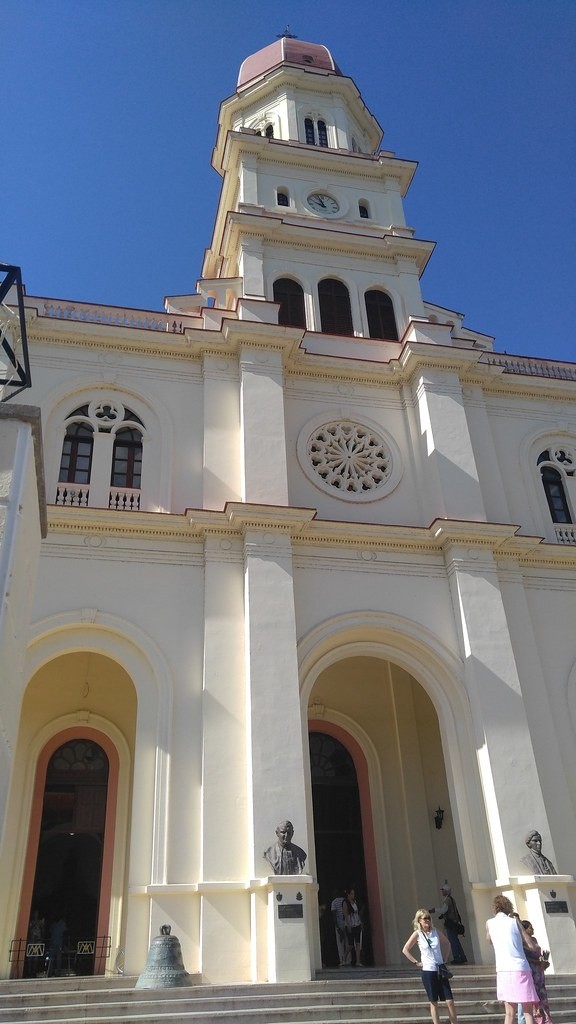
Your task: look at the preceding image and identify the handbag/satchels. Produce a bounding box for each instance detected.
[444,918,465,935]
[436,963,454,982]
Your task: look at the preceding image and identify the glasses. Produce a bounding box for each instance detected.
[419,916,431,921]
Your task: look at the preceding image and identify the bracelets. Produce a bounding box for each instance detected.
[414,961,418,964]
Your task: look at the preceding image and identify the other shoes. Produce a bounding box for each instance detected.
[450,960,462,965]
[356,962,364,967]
[459,958,467,962]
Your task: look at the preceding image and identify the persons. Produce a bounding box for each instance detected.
[319,886,364,968]
[26,910,67,976]
[263,820,307,875]
[438,884,468,965]
[485,895,552,1024]
[402,909,457,1024]
[519,829,558,875]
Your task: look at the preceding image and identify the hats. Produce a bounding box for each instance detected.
[439,884,452,895]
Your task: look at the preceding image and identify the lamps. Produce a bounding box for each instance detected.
[434,805,444,830]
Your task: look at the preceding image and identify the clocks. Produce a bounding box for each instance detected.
[307,194,339,215]
